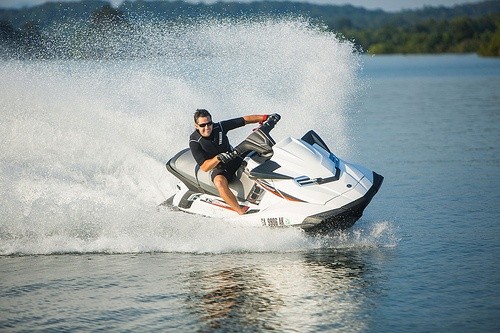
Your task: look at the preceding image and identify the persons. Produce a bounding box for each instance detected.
[189,109,271,215]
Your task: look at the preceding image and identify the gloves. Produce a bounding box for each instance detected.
[260,114,275,127]
[217,153,233,164]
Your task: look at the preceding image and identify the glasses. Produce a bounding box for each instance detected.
[195,120,212,128]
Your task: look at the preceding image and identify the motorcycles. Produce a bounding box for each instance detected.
[157,113,384,237]
